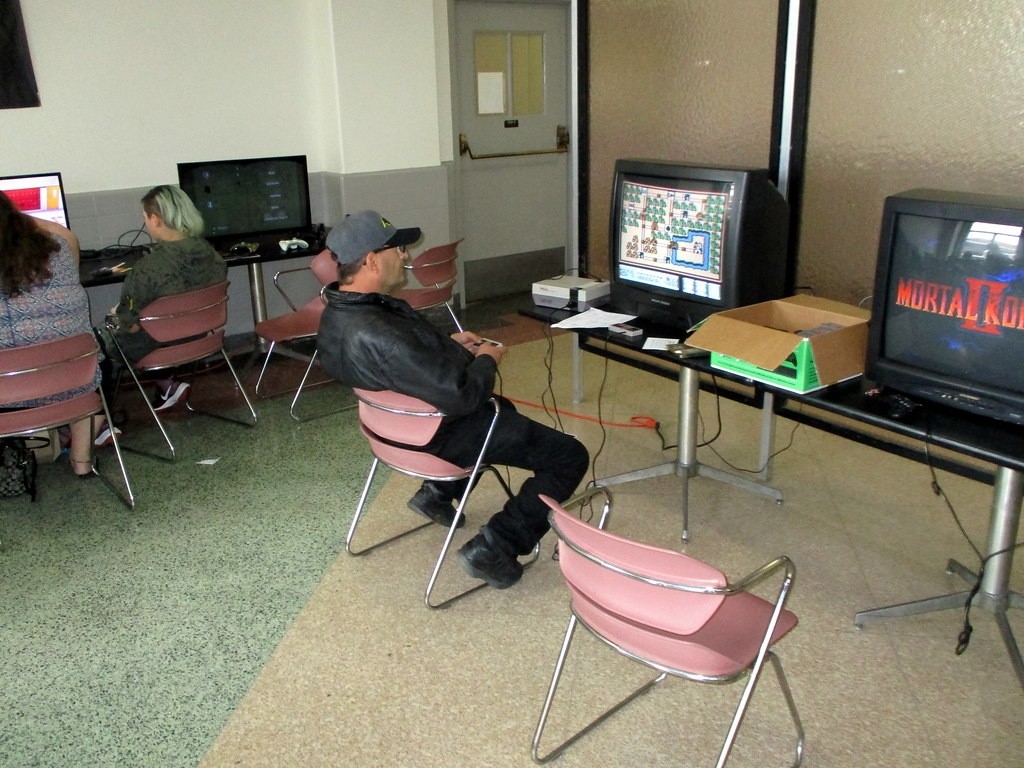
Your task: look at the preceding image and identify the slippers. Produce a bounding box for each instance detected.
[66,454,99,479]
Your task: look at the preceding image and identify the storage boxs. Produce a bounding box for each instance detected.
[684,293,872,395]
[533,275,611,313]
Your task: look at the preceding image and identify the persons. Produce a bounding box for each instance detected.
[0,188,121,480]
[316,211,589,590]
[94,183,228,448]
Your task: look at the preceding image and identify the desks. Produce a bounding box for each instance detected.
[76,227,332,386]
[516,296,1024,688]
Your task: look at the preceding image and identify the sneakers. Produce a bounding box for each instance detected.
[95,418,122,447]
[457,535,523,589]
[411,486,465,528]
[151,377,190,412]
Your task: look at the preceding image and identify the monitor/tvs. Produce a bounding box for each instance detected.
[607,158,791,335]
[864,188,1024,427]
[176,154,312,251]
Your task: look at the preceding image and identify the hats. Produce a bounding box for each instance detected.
[326,210,421,267]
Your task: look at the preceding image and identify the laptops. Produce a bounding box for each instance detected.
[0,172,70,230]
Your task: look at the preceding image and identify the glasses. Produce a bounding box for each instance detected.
[374,244,406,253]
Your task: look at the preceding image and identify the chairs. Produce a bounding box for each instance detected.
[393,236,463,334]
[254,248,359,422]
[0,332,134,552]
[530,483,805,768]
[105,280,257,463]
[344,389,540,610]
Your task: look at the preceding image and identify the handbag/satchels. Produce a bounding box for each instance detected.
[0,437,51,502]
